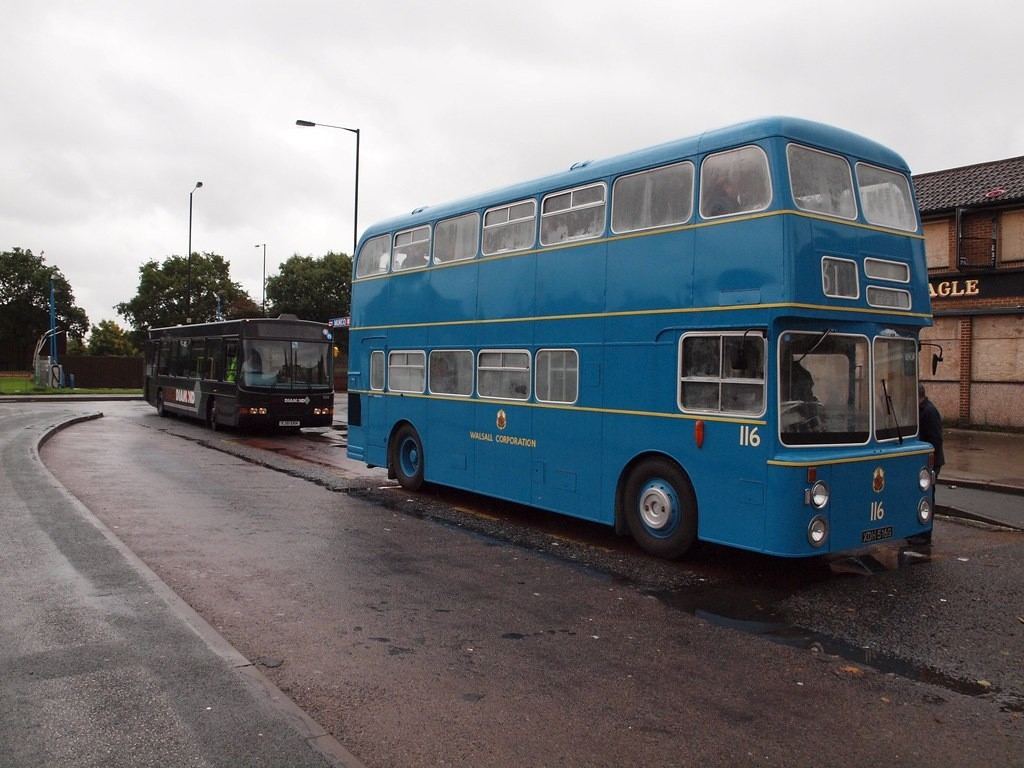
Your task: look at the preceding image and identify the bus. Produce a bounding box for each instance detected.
[347,116,943,562]
[142,314,341,433]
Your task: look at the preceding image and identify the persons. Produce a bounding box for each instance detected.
[780,348,822,431]
[431,358,455,393]
[240,350,269,377]
[480,354,528,396]
[906,384,945,546]
[704,170,741,216]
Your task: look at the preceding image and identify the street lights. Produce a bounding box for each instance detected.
[186,181,204,325]
[255,243,266,316]
[296,120,361,252]
